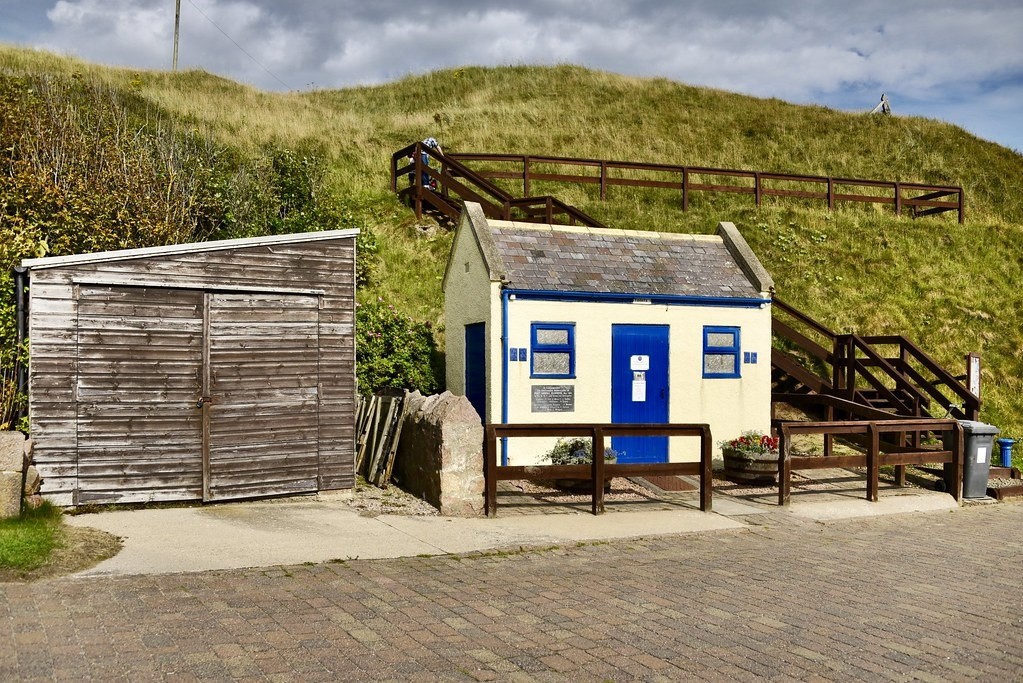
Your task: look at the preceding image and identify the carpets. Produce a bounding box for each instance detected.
[642,476,699,491]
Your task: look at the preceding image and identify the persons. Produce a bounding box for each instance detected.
[409,137,444,191]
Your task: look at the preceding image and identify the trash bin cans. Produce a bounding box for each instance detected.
[935,419,999,498]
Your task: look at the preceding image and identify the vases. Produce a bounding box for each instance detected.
[552,456,617,490]
[722,449,780,486]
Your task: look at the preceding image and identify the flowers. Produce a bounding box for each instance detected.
[714,429,778,455]
[534,437,626,466]
